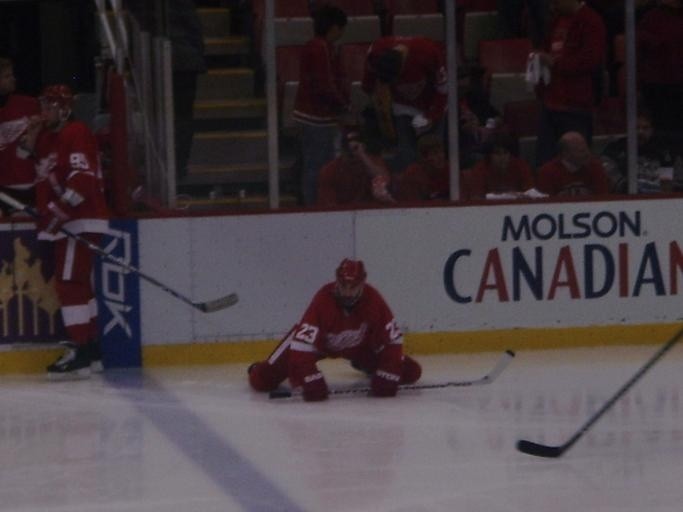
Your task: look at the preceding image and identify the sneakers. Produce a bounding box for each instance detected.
[86,336,101,363]
[46,340,90,372]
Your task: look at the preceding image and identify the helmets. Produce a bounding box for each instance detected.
[334,258,365,308]
[38,85,72,127]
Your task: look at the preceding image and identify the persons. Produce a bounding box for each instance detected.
[16,85,112,373]
[0,55,41,216]
[248,257,421,402]
[290,0,679,202]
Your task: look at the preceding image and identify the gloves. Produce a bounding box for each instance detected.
[302,372,327,401]
[370,369,400,397]
[36,201,69,236]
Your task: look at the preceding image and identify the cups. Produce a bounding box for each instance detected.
[658,168,673,194]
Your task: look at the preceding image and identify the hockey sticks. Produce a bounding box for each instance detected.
[269,350,516,399]
[515,328,683,459]
[0,194,239,314]
